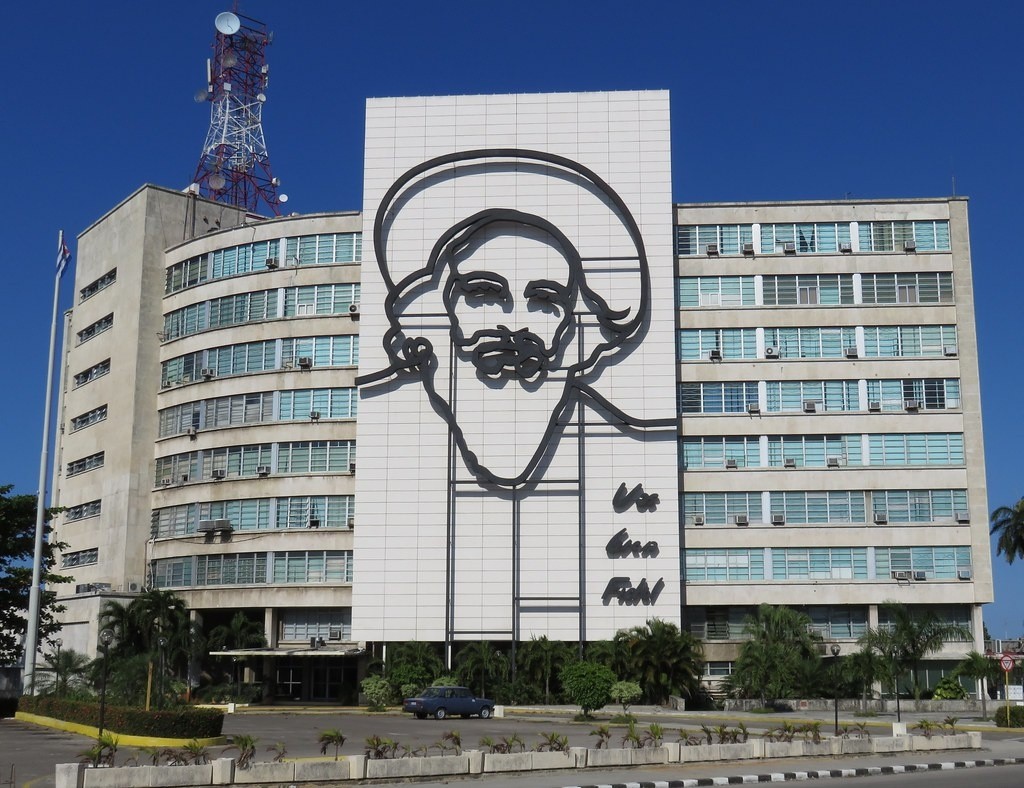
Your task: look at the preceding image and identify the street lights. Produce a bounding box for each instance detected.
[55,637,64,698]
[231,656,238,697]
[95,628,116,762]
[156,636,168,712]
[829,642,842,736]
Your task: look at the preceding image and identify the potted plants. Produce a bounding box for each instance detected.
[76,716,970,788]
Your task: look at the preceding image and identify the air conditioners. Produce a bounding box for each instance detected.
[186,426,196,435]
[349,463,356,471]
[211,469,225,478]
[298,357,312,366]
[162,380,171,387]
[902,241,916,250]
[742,243,754,254]
[256,465,271,475]
[161,478,170,486]
[839,243,851,251]
[705,244,719,255]
[310,411,318,418]
[201,368,216,377]
[329,630,340,639]
[784,242,796,253]
[265,258,279,268]
[693,345,971,580]
[348,303,360,314]
[128,581,141,592]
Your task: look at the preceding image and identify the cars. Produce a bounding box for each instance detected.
[402,686,495,720]
